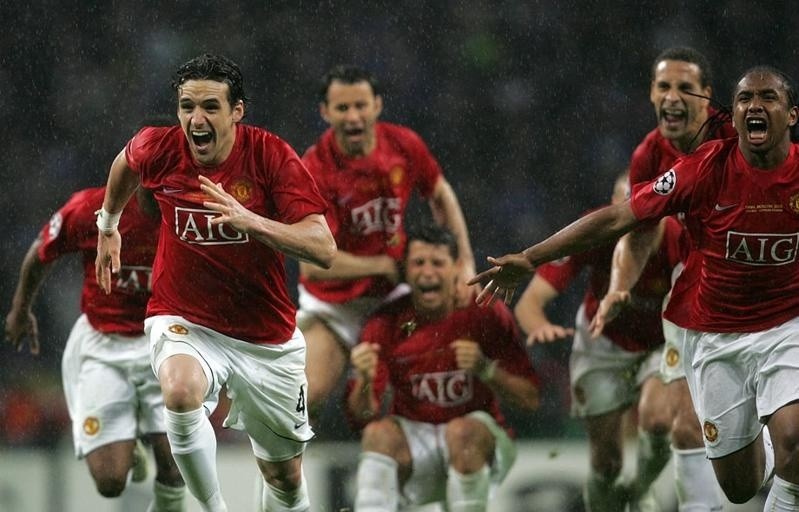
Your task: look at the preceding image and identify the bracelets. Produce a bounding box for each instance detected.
[93,206,122,232]
[480,358,498,382]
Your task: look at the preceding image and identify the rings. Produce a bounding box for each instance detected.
[494,286,505,294]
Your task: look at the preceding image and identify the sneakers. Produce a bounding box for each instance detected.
[129,433,150,484]
[618,478,659,512]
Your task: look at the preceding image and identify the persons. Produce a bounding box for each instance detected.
[341,226,541,511]
[467,67,799,511]
[288,61,483,417]
[4,112,186,512]
[586,48,737,511]
[95,55,337,511]
[511,167,685,511]
